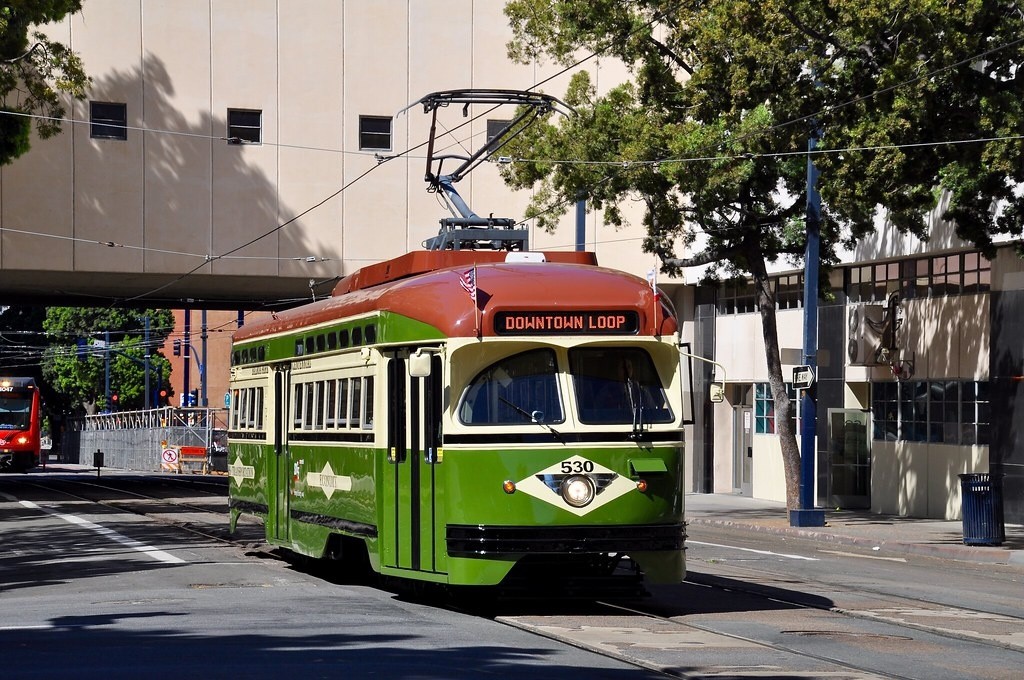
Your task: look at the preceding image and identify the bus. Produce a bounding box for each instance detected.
[0,376,40,470]
[225,88,727,602]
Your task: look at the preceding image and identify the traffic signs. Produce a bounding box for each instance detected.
[792,365,815,389]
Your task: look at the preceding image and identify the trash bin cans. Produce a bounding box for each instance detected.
[211,452,227,474]
[957,472,1006,547]
[178,446,210,475]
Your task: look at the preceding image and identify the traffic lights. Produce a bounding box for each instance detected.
[105,395,111,412]
[173,339,180,356]
[160,389,167,409]
[191,389,198,406]
[113,394,118,413]
